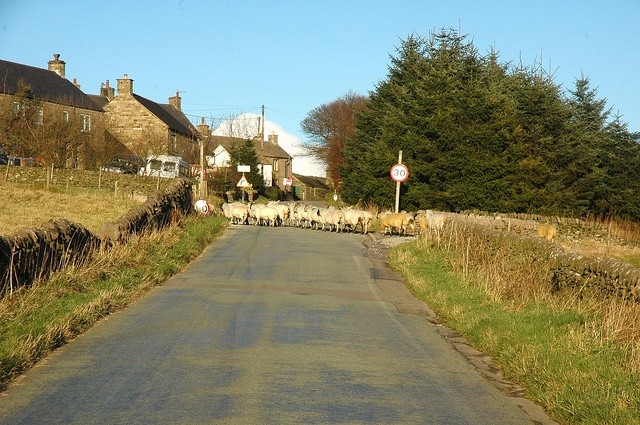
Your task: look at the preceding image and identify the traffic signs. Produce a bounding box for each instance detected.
[194,201,209,214]
[391,164,409,182]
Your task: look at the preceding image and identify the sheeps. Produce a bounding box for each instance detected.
[414,209,495,235]
[221,200,373,235]
[376,209,415,237]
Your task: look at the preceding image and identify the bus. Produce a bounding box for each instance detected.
[145,155,191,179]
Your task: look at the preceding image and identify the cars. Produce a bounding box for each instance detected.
[101,158,140,174]
